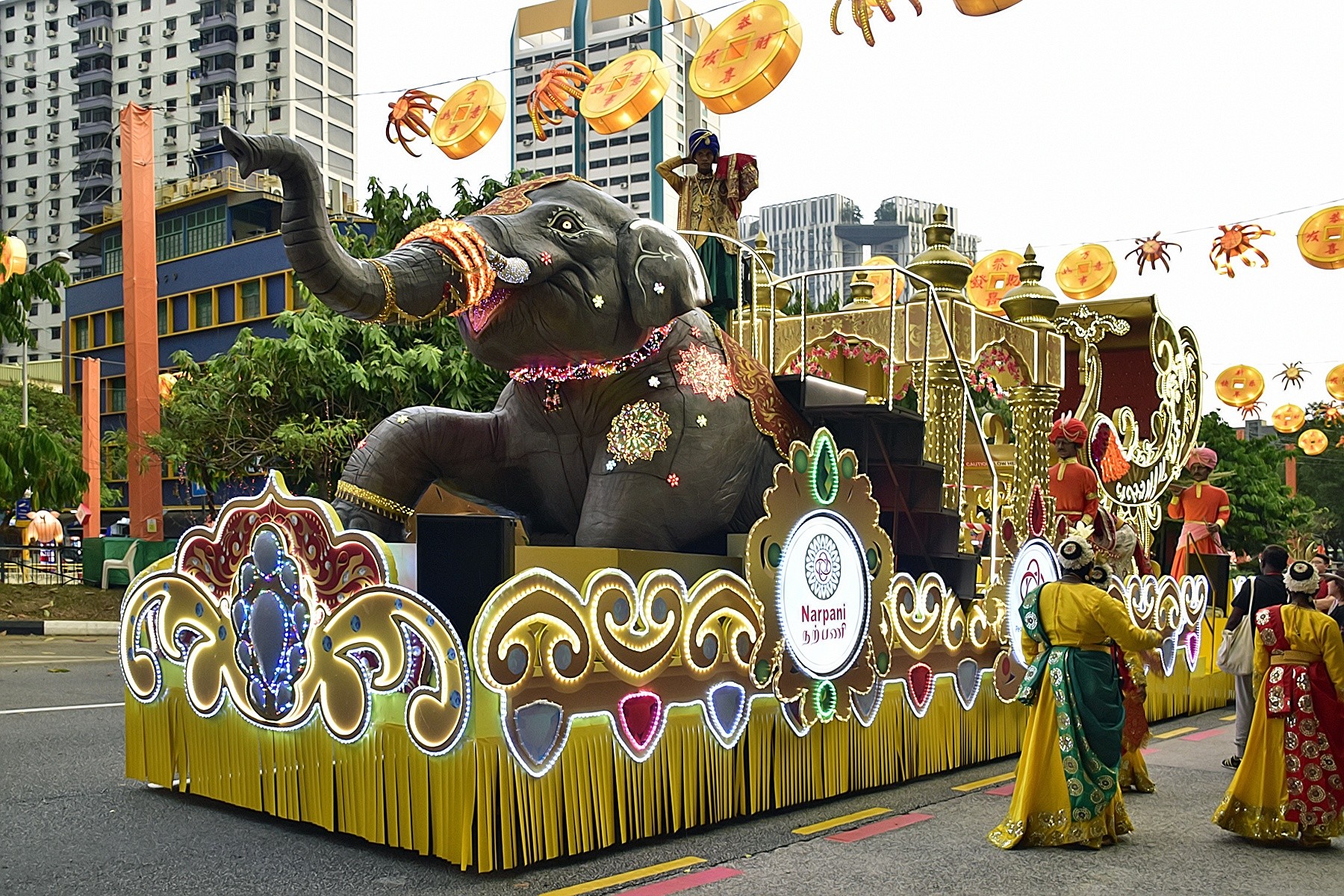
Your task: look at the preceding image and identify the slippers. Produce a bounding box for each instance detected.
[1222,756,1242,770]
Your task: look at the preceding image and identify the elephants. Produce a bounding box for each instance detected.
[213,117,814,554]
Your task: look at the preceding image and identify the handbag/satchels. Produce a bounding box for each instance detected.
[1217,614,1254,676]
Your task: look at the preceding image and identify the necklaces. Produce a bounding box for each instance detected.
[694,175,715,207]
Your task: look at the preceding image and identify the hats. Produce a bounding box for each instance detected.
[1056,535,1094,570]
[1318,564,1344,586]
[1089,562,1114,589]
[1188,447,1217,470]
[690,129,719,161]
[1282,561,1320,595]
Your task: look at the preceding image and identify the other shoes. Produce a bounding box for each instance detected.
[1299,835,1332,848]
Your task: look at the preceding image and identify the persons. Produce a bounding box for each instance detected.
[1309,553,1344,641]
[1094,507,1154,579]
[1220,545,1289,770]
[1212,560,1344,847]
[1168,447,1230,582]
[989,535,1163,849]
[656,129,759,329]
[1049,417,1099,525]
